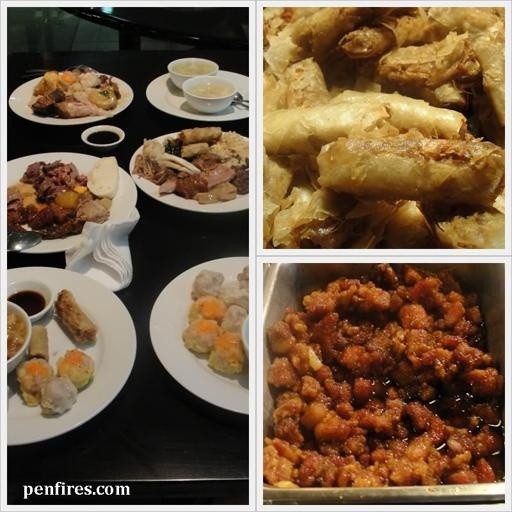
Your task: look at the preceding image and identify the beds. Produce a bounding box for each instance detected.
[6,8,249,504]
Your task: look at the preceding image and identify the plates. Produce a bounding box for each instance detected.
[81,125,125,146]
[9,74,134,125]
[150,255,248,415]
[129,131,249,214]
[7,151,136,255]
[7,280,55,323]
[7,266,136,448]
[146,69,249,121]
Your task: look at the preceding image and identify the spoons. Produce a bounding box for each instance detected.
[232,91,249,105]
[7,231,44,253]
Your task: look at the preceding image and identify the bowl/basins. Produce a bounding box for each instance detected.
[7,301,33,375]
[168,57,219,90]
[182,75,235,114]
